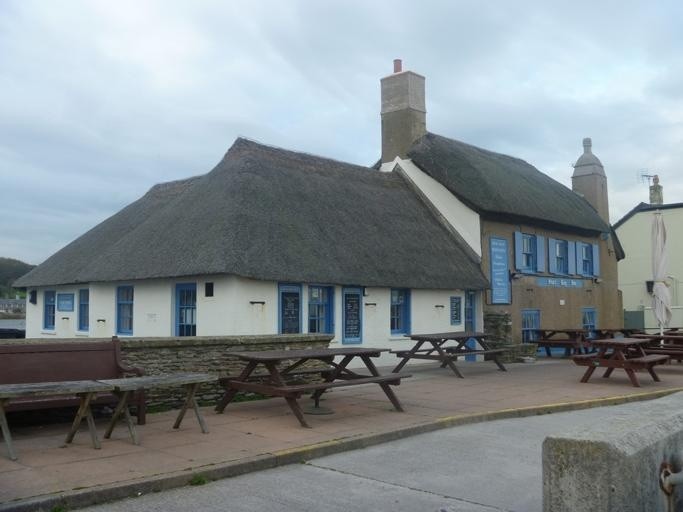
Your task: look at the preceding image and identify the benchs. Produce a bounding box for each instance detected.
[646,339,682,363]
[389,346,458,373]
[625,354,668,387]
[446,348,507,379]
[276,372,411,428]
[574,351,614,382]
[215,365,336,414]
[0,335,145,425]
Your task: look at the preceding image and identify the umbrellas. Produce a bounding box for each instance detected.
[650,211,672,348]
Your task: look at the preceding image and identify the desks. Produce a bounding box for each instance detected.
[524,328,590,339]
[96,372,219,445]
[0,379,114,461]
[217,346,391,399]
[404,332,493,355]
[590,340,650,369]
